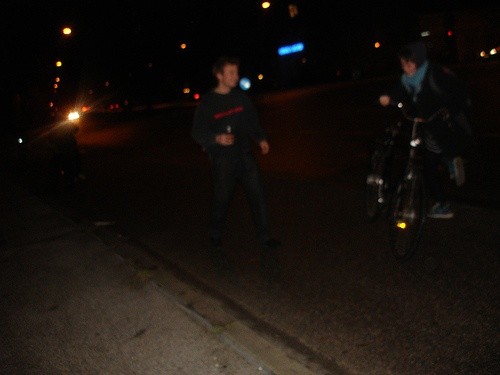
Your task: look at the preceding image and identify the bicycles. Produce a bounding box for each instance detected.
[363,100,455,262]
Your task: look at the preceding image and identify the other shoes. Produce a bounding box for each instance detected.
[426,201,454,220]
[447,157,466,187]
[259,239,280,246]
[211,237,222,247]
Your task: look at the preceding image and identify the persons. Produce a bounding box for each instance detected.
[376,45,468,219]
[191,56,282,252]
